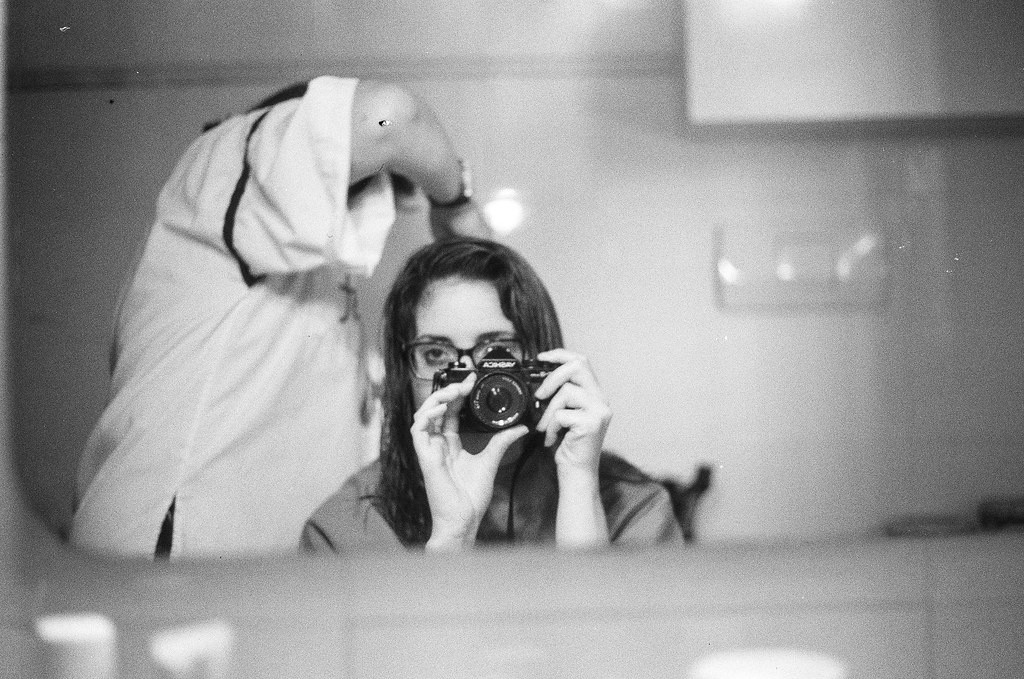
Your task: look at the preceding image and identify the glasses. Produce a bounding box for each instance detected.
[400,338,527,382]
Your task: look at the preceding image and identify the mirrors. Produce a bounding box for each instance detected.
[6,2,1024,560]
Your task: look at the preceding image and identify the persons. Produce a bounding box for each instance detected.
[71,75,498,561]
[299,237,688,553]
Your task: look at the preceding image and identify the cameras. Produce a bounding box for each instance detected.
[433,350,571,432]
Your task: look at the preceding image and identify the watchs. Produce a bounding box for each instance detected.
[428,160,473,207]
